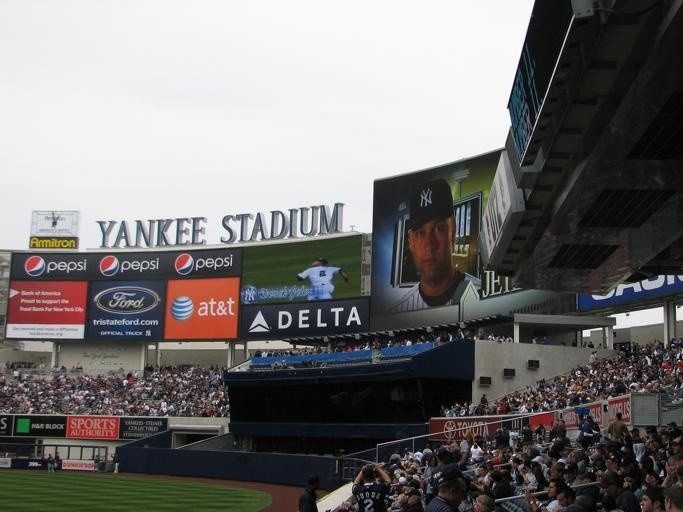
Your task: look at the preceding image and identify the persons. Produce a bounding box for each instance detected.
[0,359,233,419]
[51,451,60,470]
[111,453,121,474]
[46,452,56,473]
[296,256,349,302]
[385,176,481,314]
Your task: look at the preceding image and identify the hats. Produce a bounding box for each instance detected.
[408,177,456,229]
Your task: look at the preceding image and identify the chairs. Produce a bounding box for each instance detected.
[0,369,230,419]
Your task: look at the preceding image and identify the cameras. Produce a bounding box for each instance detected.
[367,464,373,468]
